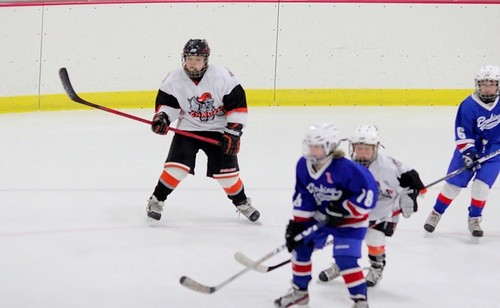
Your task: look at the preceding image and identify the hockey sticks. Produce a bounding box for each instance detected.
[234,209,403,273]
[58,66,222,145]
[424,149,500,188]
[180,219,328,295]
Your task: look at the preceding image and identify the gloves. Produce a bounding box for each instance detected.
[222,122,244,156]
[286,219,304,253]
[397,187,419,217]
[324,202,348,220]
[462,152,481,173]
[151,112,169,135]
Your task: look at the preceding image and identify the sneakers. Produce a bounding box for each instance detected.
[274,287,309,308]
[352,300,369,308]
[468,219,483,237]
[236,197,261,224]
[366,264,383,286]
[146,195,165,223]
[423,211,442,232]
[319,263,340,281]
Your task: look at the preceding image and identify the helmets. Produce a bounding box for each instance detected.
[348,122,381,166]
[180,39,210,78]
[474,64,500,102]
[305,120,340,162]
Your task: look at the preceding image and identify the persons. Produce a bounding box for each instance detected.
[273,124,379,308]
[423,65,500,237]
[319,122,415,287]
[146,39,261,222]
